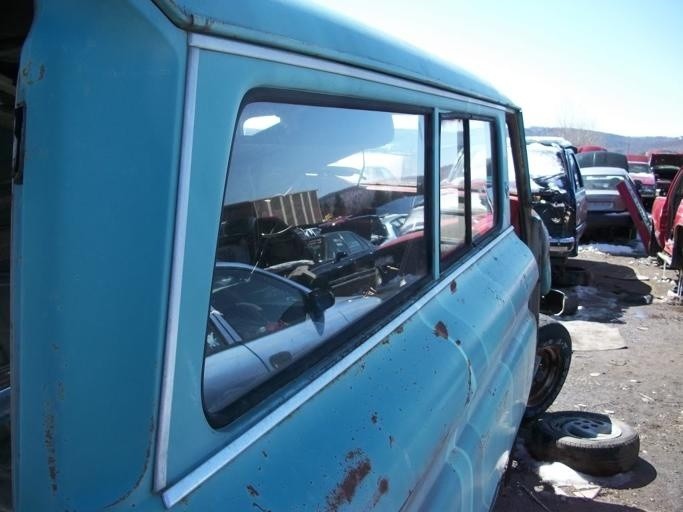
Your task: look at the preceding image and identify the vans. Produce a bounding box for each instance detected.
[0,1,570,512]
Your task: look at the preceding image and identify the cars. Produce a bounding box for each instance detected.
[203,262,384,413]
[439,136,682,306]
[215,191,495,300]
